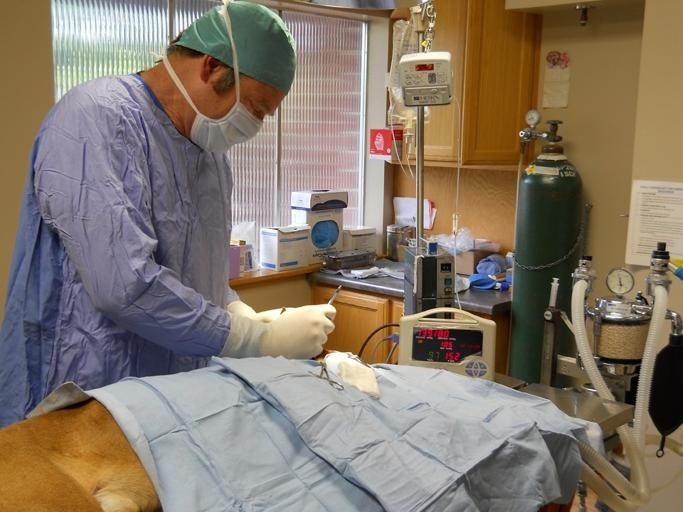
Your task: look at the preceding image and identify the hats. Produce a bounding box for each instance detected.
[171,1,298,96]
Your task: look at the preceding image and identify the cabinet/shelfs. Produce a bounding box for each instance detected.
[392,1,541,170]
[228,249,510,382]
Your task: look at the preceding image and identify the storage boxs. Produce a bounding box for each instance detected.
[260,187,377,271]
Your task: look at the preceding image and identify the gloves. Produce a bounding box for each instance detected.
[216,300,337,359]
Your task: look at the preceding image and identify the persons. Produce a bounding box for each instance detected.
[0,0,340,429]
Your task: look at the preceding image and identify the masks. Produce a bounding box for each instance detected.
[188,99,265,155]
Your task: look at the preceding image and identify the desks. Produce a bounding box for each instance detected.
[495,360,635,436]
